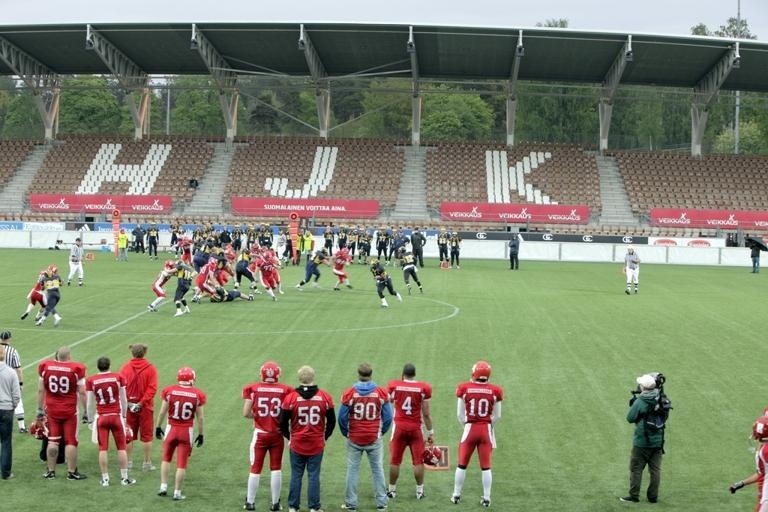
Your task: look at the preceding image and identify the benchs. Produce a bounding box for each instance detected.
[0,133,768,237]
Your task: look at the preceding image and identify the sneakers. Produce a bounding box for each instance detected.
[172,495,186,500]
[191,287,202,304]
[626,287,639,296]
[184,306,190,313]
[156,487,168,496]
[234,285,285,302]
[19,426,29,433]
[173,308,183,318]
[296,283,353,291]
[20,313,62,327]
[148,304,157,312]
[408,285,423,296]
[42,461,159,486]
[380,294,402,307]
[619,496,639,503]
[243,490,492,511]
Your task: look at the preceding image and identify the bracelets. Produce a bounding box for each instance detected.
[428,430,434,434]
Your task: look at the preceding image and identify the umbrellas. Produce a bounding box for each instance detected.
[744,237,768,252]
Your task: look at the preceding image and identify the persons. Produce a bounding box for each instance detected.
[729,416,768,512]
[619,374,671,504]
[242,361,295,512]
[189,177,199,188]
[509,235,519,270]
[0,330,208,500]
[450,360,503,507]
[22,223,463,328]
[623,248,640,295]
[386,363,434,499]
[750,242,760,273]
[338,364,393,511]
[279,366,336,512]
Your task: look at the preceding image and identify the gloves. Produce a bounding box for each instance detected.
[156,427,164,440]
[81,414,88,423]
[194,434,204,447]
[127,403,141,413]
[728,480,744,493]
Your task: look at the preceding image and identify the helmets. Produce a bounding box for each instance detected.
[398,247,405,253]
[47,264,58,277]
[342,247,350,253]
[210,294,223,302]
[260,361,281,382]
[370,258,379,266]
[421,440,441,465]
[748,415,768,449]
[30,422,50,439]
[176,367,196,384]
[124,424,134,444]
[319,248,328,254]
[165,235,275,271]
[472,361,491,381]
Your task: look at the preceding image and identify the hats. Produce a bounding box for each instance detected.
[0,330,12,340]
[636,374,656,389]
